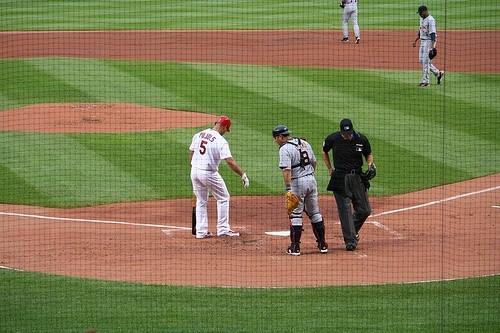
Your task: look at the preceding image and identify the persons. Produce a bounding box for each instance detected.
[340,0,360,44]
[189,115,250,238]
[322,118,376,251]
[413,5,444,87]
[273,125,328,256]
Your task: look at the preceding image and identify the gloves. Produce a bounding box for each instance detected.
[241,173,249,188]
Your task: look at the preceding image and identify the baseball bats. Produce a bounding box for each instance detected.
[191,191,196,236]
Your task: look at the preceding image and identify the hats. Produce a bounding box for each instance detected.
[216,116,231,132]
[340,119,354,135]
[417,6,427,13]
[273,125,292,135]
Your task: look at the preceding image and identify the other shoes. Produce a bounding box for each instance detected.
[341,37,349,42]
[355,36,360,44]
[346,244,356,251]
[419,82,429,86]
[437,71,443,85]
[355,232,360,241]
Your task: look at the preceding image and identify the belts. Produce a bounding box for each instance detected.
[345,171,354,174]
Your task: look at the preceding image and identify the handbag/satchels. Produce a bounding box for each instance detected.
[357,169,370,190]
[326,171,346,194]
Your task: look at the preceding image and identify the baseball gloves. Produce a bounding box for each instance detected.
[357,166,377,188]
[284,192,301,215]
[428,48,438,59]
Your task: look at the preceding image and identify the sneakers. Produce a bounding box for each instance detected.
[320,242,328,253]
[218,230,240,237]
[285,247,301,255]
[204,231,212,238]
